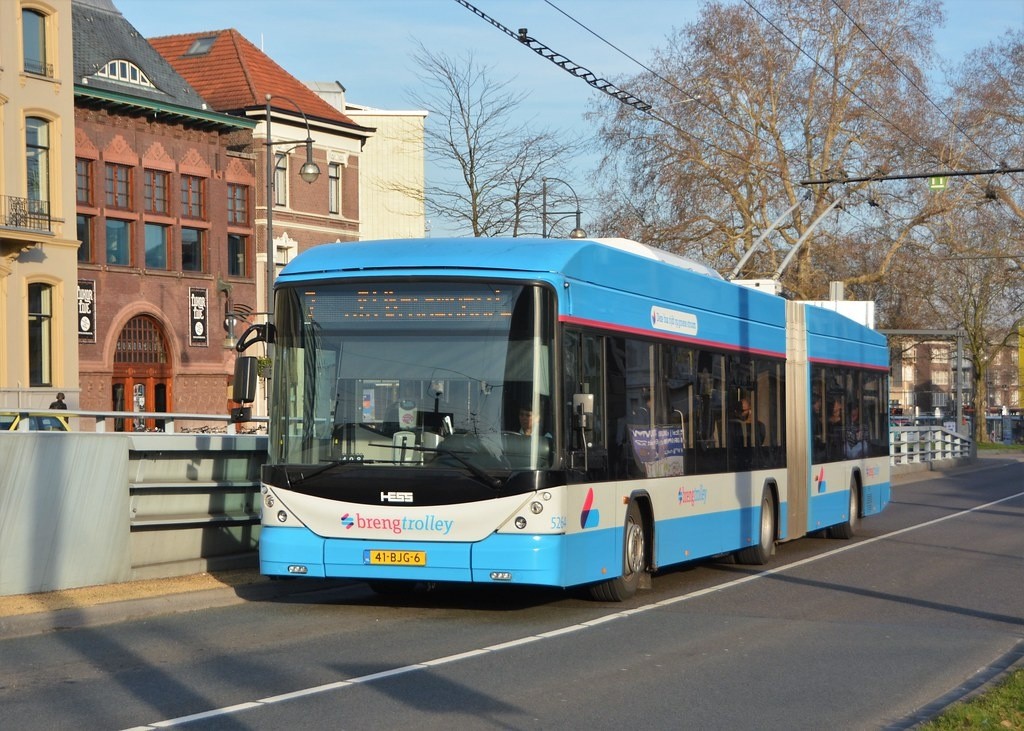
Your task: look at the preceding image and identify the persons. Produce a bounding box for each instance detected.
[516,402,554,439]
[811,393,870,458]
[48,392,69,424]
[618,376,767,450]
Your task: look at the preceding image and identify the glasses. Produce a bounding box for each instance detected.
[737,408,751,413]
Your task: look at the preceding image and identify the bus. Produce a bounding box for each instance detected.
[231,236,902,591]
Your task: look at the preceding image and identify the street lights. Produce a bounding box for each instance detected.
[541,177,593,245]
[261,93,323,424]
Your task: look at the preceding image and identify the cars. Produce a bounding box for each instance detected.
[0,411,77,432]
